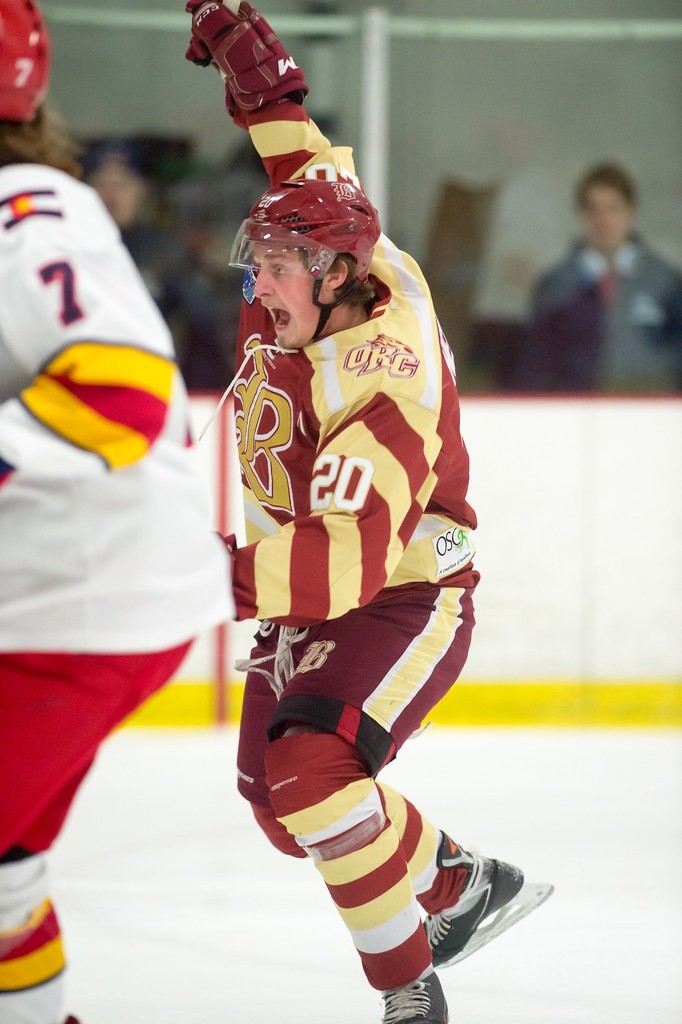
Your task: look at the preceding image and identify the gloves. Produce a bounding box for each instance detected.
[185,0,311,119]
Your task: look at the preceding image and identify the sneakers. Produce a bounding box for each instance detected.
[422,850,554,969]
[381,963,449,1024]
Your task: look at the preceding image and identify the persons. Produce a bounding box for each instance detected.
[0,0,235,1023]
[531,163,682,395]
[83,147,234,392]
[182,0,554,1024]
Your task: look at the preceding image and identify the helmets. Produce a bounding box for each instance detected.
[0,0,50,121]
[242,178,382,284]
